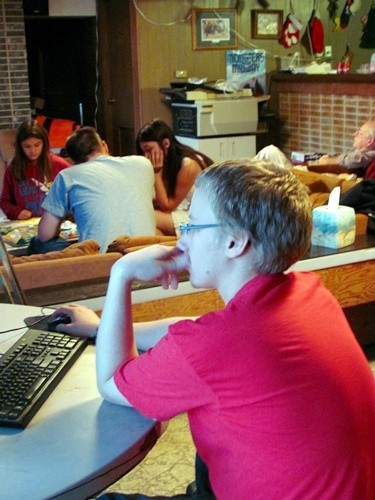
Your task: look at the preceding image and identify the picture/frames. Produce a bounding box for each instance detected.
[252,9,283,38]
[190,8,237,51]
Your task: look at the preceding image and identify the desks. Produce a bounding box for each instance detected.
[0,304,168,500]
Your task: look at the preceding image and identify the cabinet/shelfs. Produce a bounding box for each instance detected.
[175,136,257,164]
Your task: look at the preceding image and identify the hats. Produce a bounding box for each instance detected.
[300,15,324,54]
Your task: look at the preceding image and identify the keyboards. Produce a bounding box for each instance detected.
[0,321,90,428]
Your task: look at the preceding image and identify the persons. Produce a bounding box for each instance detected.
[136,117,216,237]
[305,178,375,215]
[27,128,156,254]
[52,159,375,500]
[0,121,72,220]
[256,121,375,175]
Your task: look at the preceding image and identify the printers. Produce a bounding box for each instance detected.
[170,97,258,138]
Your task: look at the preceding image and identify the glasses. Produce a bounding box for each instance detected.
[179,223,219,236]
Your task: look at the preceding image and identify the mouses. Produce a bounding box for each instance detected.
[47,315,71,332]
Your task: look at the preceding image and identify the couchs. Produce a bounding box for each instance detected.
[0,166,364,293]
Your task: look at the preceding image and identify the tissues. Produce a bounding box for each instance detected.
[309,186,357,250]
